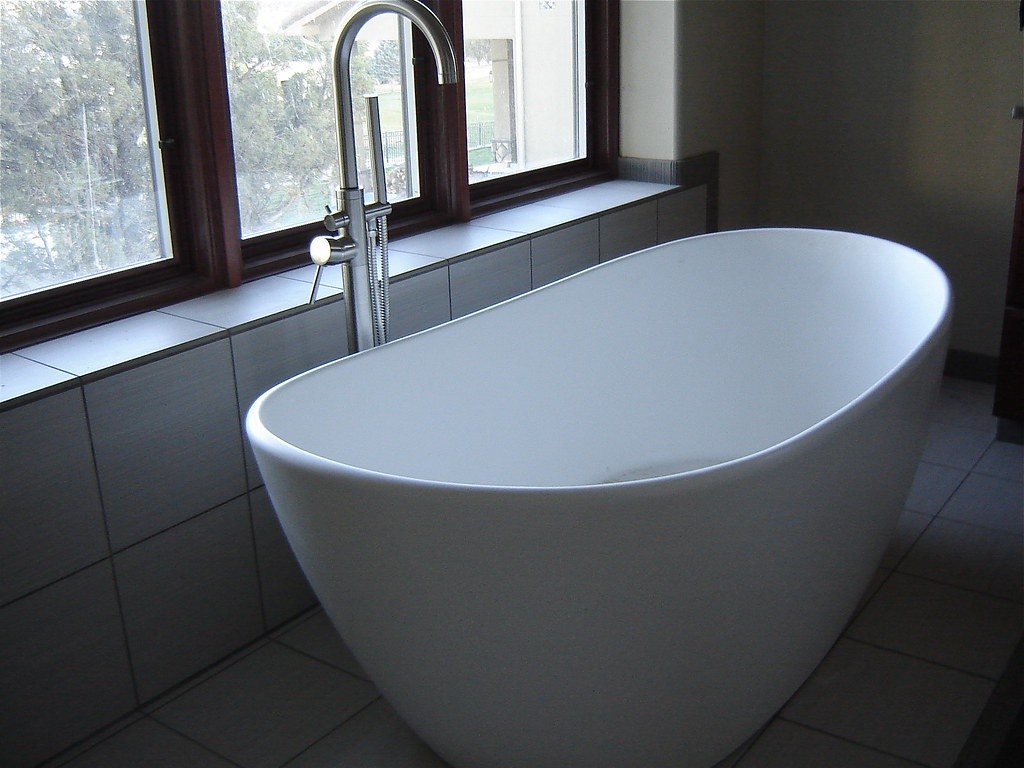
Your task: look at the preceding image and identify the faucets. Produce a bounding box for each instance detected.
[334,1,459,187]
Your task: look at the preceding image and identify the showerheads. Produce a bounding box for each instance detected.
[363,93,387,203]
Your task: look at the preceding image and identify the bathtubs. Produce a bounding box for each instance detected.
[247,224,955,766]
